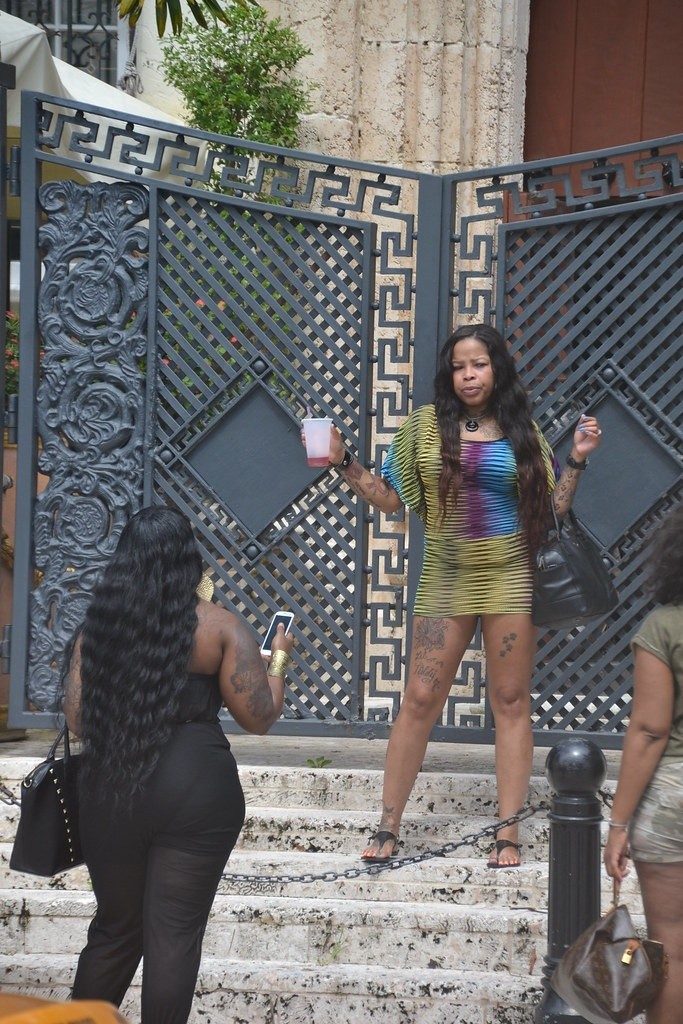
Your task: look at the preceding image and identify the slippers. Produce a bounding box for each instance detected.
[486,840,522,868]
[361,831,398,862]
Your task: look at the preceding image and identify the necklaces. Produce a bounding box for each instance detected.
[461,412,492,431]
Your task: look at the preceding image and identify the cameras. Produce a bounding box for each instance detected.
[260,611,295,656]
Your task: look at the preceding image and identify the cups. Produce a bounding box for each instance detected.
[301,418,333,467]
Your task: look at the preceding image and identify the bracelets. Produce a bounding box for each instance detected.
[267,650,290,679]
[609,822,630,830]
[334,448,351,472]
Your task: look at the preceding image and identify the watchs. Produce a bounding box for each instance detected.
[567,455,589,471]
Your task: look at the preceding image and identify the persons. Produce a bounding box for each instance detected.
[300,323,601,869]
[604,502,683,1024]
[56,506,293,1023]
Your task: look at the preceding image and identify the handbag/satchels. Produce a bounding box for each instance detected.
[530,491,621,631]
[8,718,84,878]
[550,860,668,1024]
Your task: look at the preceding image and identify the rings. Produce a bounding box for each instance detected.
[598,429,602,435]
